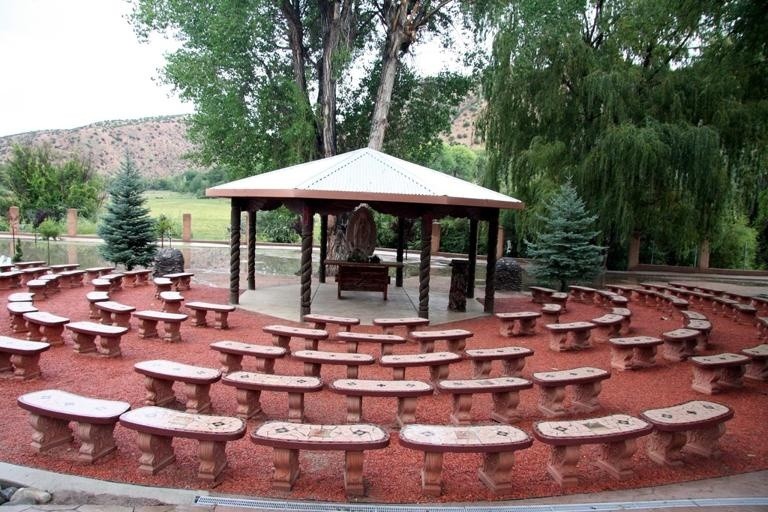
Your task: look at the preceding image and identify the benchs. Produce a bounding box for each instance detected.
[328,377,435,426]
[377,351,463,380]
[209,339,286,373]
[660,328,700,361]
[0,256,237,383]
[303,313,360,332]
[531,414,654,488]
[687,352,751,395]
[494,256,768,353]
[335,332,407,355]
[609,335,664,372]
[134,359,222,414]
[465,345,535,379]
[640,399,735,468]
[741,344,768,380]
[399,424,534,496]
[407,328,473,354]
[16,389,132,465]
[261,324,329,356]
[119,405,246,483]
[294,349,375,379]
[531,366,611,418]
[249,420,391,497]
[223,370,324,425]
[436,376,534,426]
[376,317,430,335]
